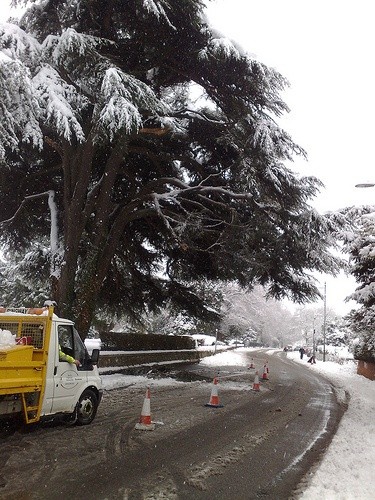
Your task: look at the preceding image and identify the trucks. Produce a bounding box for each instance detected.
[1,304,103,425]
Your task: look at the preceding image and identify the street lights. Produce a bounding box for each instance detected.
[355,181,375,190]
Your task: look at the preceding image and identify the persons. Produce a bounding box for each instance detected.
[58,344,80,367]
[299,347,305,359]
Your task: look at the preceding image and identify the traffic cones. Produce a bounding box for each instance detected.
[250,370,261,392]
[265,359,270,373]
[261,365,269,380]
[204,379,224,407]
[247,356,256,370]
[134,385,155,431]
